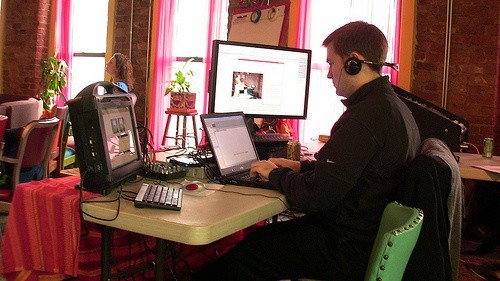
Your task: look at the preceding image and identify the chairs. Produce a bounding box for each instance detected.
[49,104,69,177]
[0,117,61,189]
[365,200,424,281]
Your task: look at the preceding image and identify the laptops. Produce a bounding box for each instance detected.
[199,111,275,189]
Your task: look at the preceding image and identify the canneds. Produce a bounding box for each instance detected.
[482,137,495,159]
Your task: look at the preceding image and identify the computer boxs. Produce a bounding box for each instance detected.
[393,84,471,152]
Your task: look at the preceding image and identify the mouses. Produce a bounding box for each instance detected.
[181,180,206,192]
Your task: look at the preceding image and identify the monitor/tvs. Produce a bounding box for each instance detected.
[207,40,311,119]
[65,80,146,196]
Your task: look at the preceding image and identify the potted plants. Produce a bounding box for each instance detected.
[164,58,196,111]
[38,55,69,121]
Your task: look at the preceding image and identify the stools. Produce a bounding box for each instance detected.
[161,111,199,149]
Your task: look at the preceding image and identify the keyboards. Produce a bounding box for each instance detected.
[252,133,291,148]
[134,182,184,211]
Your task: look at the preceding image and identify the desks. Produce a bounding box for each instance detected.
[13,147,292,280]
[0,93,44,129]
[452,151,500,183]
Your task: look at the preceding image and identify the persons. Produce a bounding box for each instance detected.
[161,19,424,281]
[105,51,135,92]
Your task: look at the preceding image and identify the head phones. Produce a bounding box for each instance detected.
[344,57,400,76]
